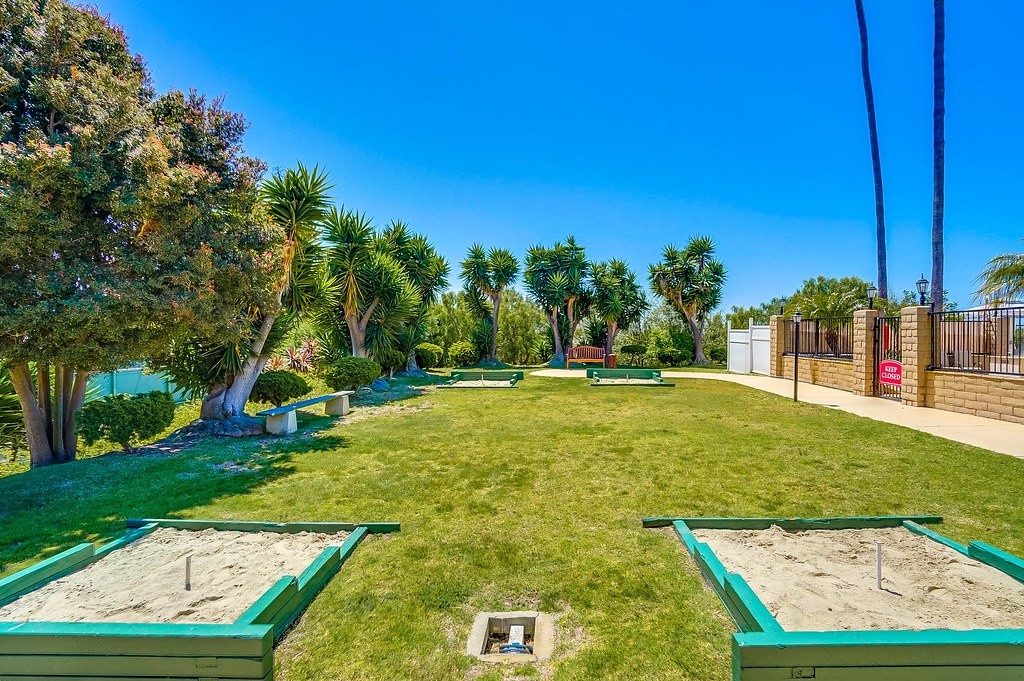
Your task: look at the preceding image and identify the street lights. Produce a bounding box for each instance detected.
[792,310,803,402]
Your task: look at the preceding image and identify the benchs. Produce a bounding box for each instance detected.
[566,346,606,368]
[256,390,355,436]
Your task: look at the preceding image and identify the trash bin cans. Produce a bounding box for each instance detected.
[608,354,617,369]
